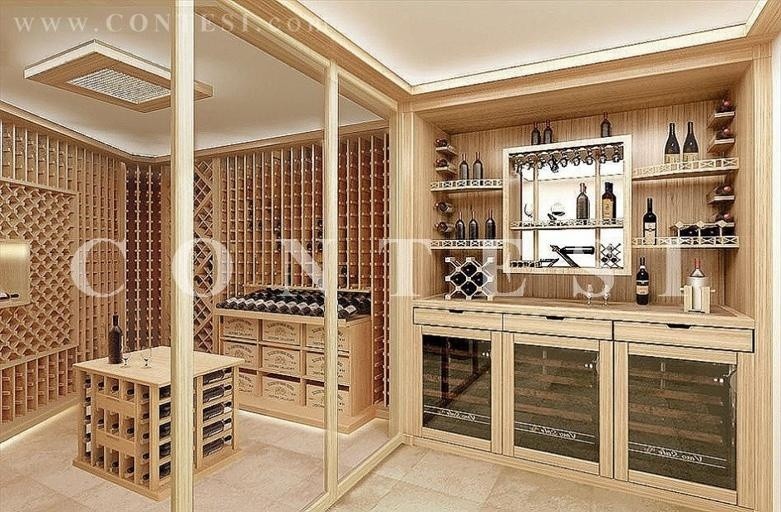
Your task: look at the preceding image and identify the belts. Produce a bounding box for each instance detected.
[120,344,133,370]
[550,203,565,221]
[141,342,153,368]
[524,202,534,227]
[511,144,621,173]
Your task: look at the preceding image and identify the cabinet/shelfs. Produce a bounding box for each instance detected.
[108,313,124,366]
[436,158,458,174]
[712,99,733,116]
[213,286,373,320]
[485,206,498,241]
[434,220,457,234]
[642,197,658,245]
[611,183,616,223]
[454,210,466,242]
[708,210,732,223]
[434,201,458,215]
[435,138,458,153]
[709,128,733,148]
[544,119,553,144]
[682,121,700,169]
[552,245,596,256]
[689,256,706,277]
[584,186,590,221]
[664,122,681,170]
[673,228,736,244]
[601,111,611,139]
[82,371,149,488]
[458,152,469,186]
[602,182,617,223]
[450,263,487,295]
[532,120,541,145]
[0,289,21,303]
[576,182,591,227]
[706,184,732,202]
[634,256,650,306]
[469,209,479,242]
[159,365,234,479]
[473,152,483,186]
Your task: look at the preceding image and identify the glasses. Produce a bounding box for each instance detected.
[24,40,213,113]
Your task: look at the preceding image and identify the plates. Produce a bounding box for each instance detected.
[71,346,248,502]
[211,285,376,434]
[397,39,774,512]
